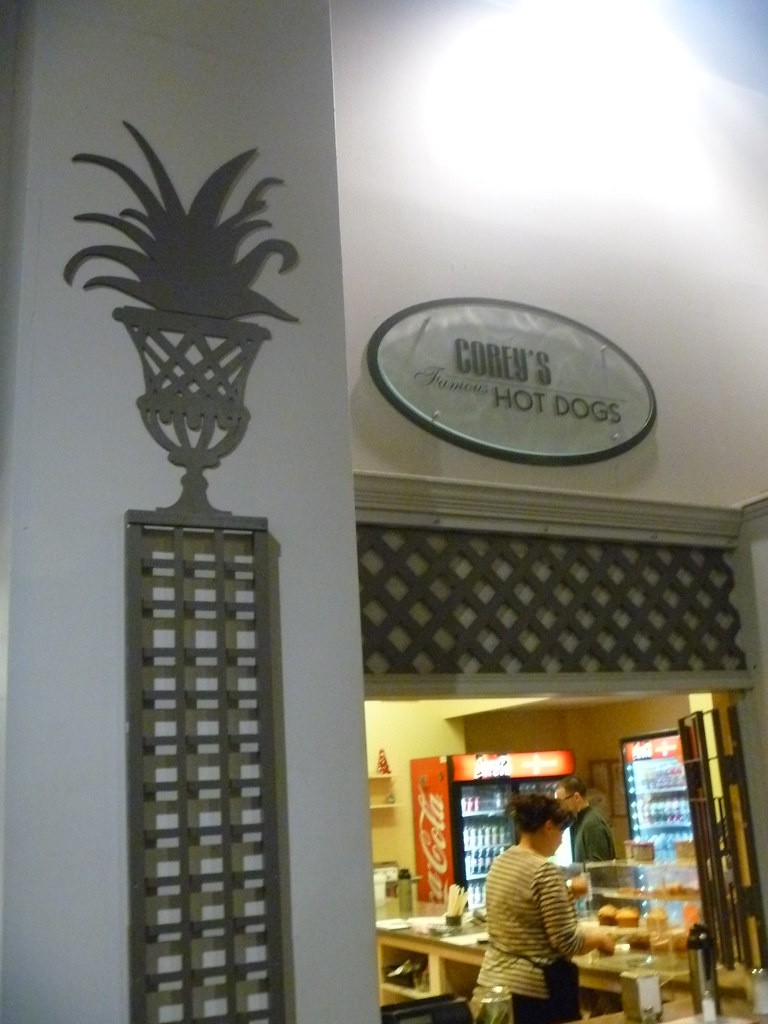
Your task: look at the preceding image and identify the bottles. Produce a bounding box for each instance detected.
[634,767,687,789]
[460,816,512,849]
[467,882,487,906]
[464,846,505,875]
[632,829,695,862]
[397,867,413,913]
[640,1005,657,1024]
[374,867,387,903]
[518,781,564,798]
[460,786,504,814]
[634,797,692,825]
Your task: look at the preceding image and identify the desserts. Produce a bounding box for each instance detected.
[614,906,641,928]
[597,904,620,927]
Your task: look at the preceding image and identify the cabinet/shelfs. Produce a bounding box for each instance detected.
[369,774,403,809]
[376,916,693,1024]
[585,861,705,937]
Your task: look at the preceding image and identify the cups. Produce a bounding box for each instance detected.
[472,984,515,1024]
[445,915,461,927]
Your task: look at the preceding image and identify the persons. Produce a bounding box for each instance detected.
[471,794,615,1024]
[555,775,621,910]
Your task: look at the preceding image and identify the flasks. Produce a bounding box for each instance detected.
[686,922,722,1019]
